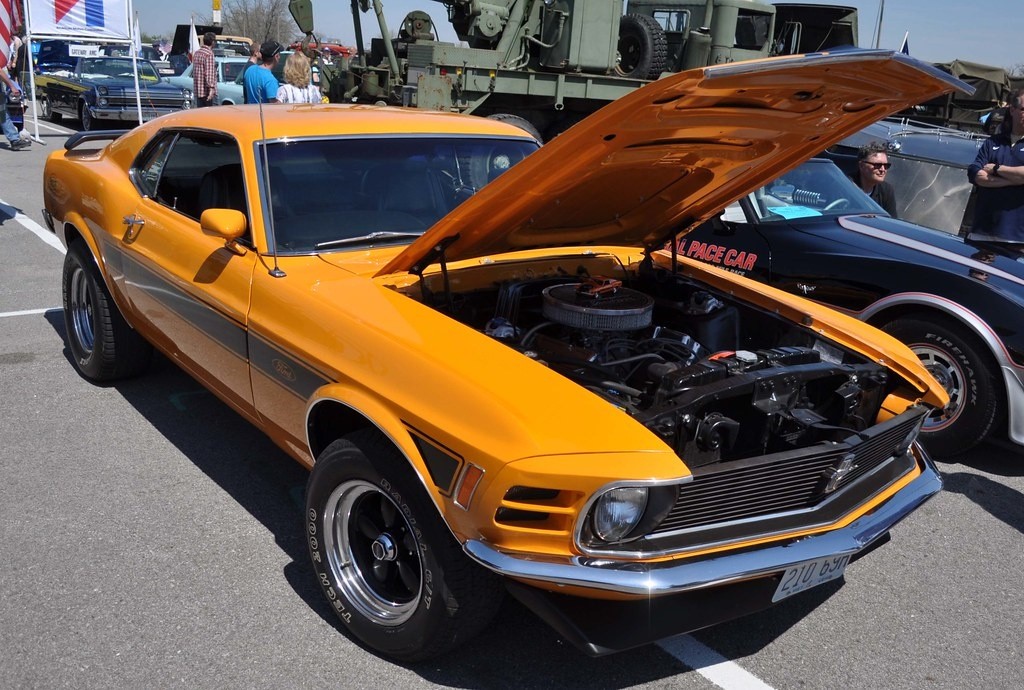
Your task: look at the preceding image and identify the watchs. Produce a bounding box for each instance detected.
[993,164,1000,175]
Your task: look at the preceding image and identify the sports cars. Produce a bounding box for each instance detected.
[40,36,981,668]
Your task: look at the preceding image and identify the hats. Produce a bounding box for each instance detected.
[323,47,330,51]
[260,41,284,56]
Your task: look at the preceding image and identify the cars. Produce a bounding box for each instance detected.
[660,156,1024,461]
[14,35,354,131]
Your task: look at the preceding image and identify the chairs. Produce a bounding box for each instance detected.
[200,163,296,225]
[356,158,448,215]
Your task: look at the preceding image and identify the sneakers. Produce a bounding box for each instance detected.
[10,139,31,150]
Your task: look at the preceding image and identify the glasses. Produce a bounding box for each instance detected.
[272,41,280,55]
[862,160,892,170]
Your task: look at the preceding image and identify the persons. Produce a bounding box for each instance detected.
[152,41,162,59]
[311,47,333,66]
[111,50,120,57]
[193,32,217,108]
[242,43,262,104]
[847,141,897,218]
[957,88,1024,260]
[0,35,32,151]
[275,52,322,103]
[963,237,1022,281]
[244,40,284,104]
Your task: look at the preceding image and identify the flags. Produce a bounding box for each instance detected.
[0,0,23,71]
[901,40,909,55]
[129,19,141,57]
[188,19,200,68]
[161,40,172,53]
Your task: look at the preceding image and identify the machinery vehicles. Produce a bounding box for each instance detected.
[287,0,862,209]
